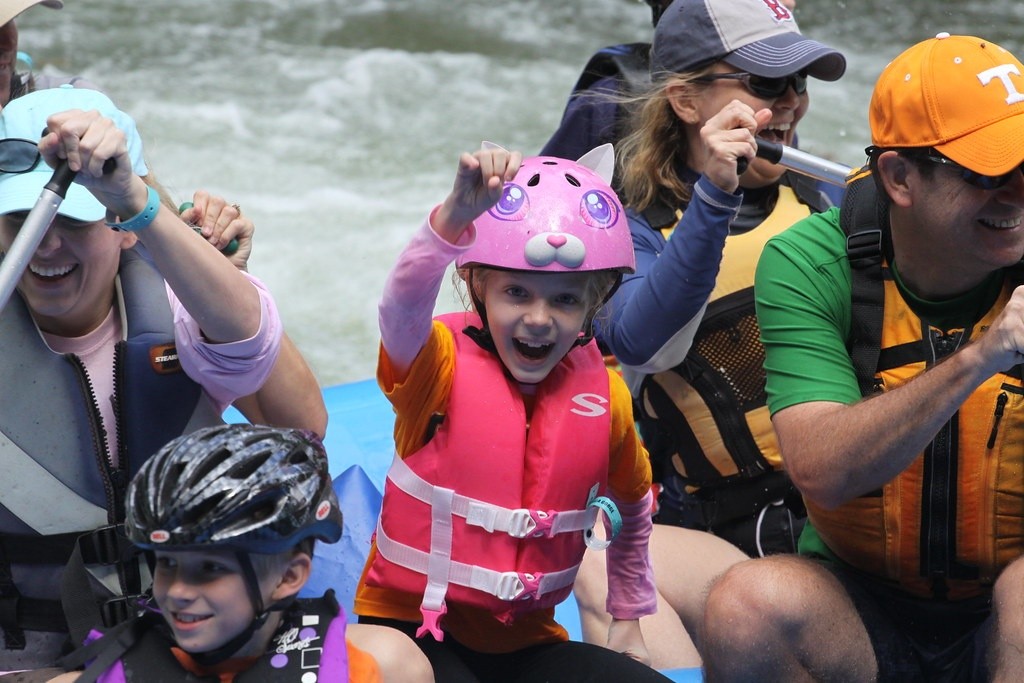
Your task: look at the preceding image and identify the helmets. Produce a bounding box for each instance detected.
[123,424,343,552]
[456,140,636,276]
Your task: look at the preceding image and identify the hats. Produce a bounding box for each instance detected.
[0,0,63,27]
[649,0,846,84]
[0,84,149,222]
[868,32,1024,177]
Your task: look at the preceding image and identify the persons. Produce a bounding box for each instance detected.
[78,422,380,683]
[571,0,848,682]
[0,19,254,274]
[0,84,436,683]
[537,1,674,208]
[702,30,1023,683]
[353,145,676,682]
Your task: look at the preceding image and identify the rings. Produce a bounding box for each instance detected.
[231,204,242,219]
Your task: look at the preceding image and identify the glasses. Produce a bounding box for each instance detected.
[864,146,1024,189]
[0,138,41,174]
[685,71,807,98]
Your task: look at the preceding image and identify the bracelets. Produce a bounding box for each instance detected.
[102,185,160,234]
